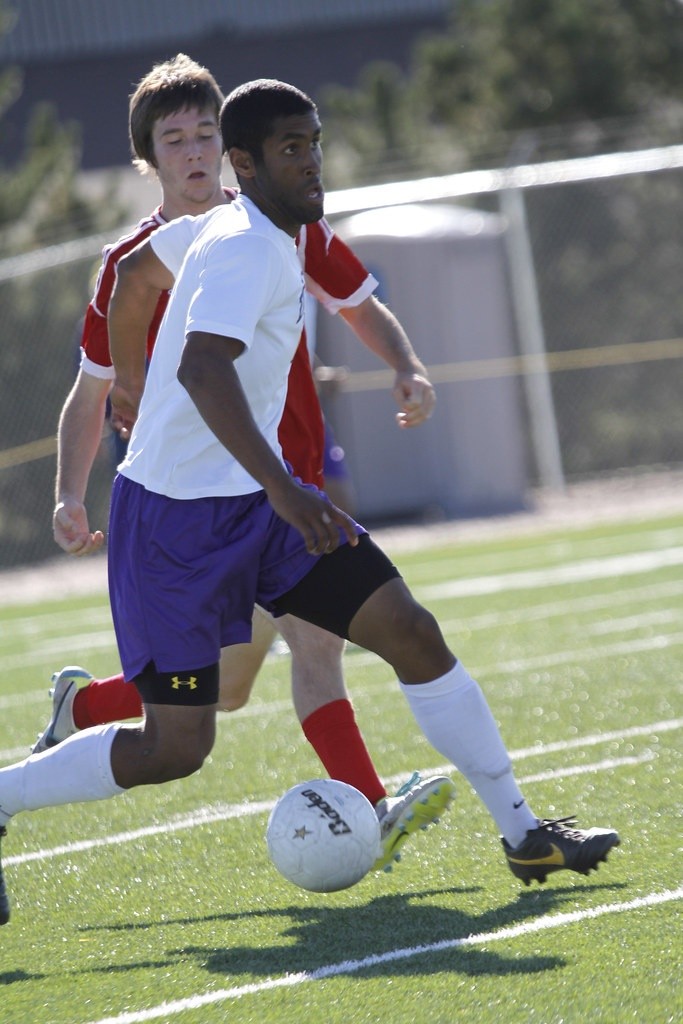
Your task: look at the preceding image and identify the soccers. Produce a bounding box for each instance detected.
[266,778,383,894]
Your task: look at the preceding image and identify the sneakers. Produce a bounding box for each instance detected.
[0,826,10,926]
[30,666,96,754]
[499,816,622,886]
[370,771,454,873]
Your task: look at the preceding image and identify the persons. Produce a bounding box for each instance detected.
[28,55,457,873]
[0,80,617,925]
